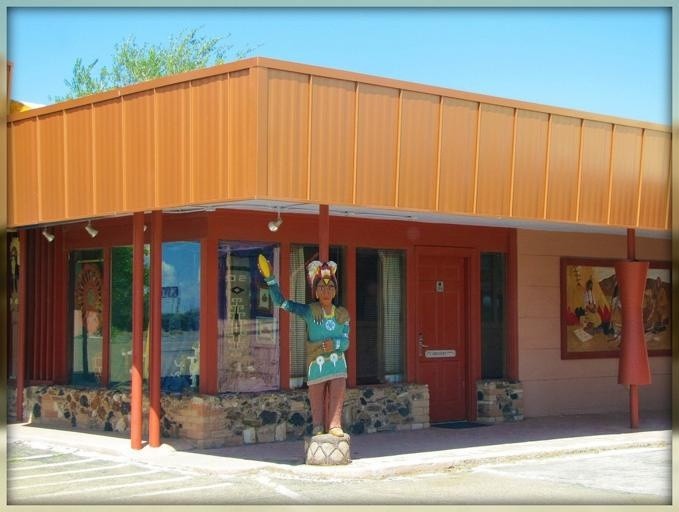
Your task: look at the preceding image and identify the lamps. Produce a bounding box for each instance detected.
[268,212,285,233]
[83,217,102,241]
[40,225,58,243]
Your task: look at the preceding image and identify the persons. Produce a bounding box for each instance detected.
[255,253,352,437]
[583,280,601,331]
[646,278,672,332]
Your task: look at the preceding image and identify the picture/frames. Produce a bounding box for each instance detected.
[254,281,273,313]
[559,258,674,361]
[255,315,276,344]
